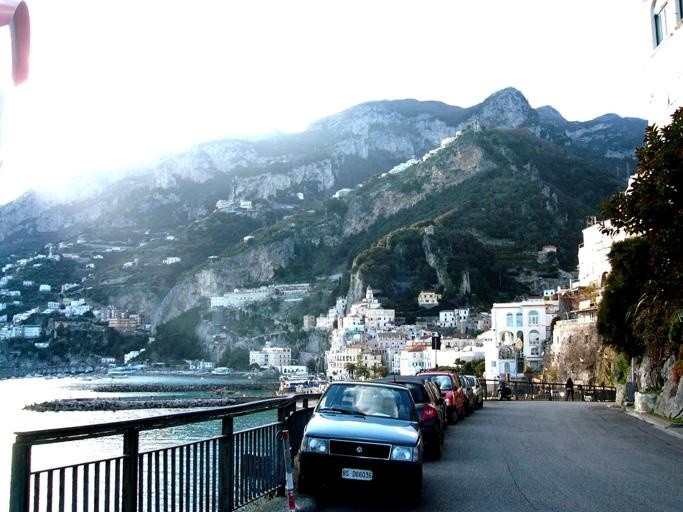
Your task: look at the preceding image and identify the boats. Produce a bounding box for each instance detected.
[109,371,128,379]
[211,366,232,375]
[271,373,329,408]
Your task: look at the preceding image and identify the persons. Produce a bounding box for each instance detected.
[565,377,575,401]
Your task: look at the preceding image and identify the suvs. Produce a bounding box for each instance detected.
[415,367,465,424]
[366,375,447,459]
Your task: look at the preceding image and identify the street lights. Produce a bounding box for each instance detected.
[579,355,585,384]
[603,351,612,387]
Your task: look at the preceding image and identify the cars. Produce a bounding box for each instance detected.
[457,372,475,414]
[465,374,483,409]
[292,378,425,504]
